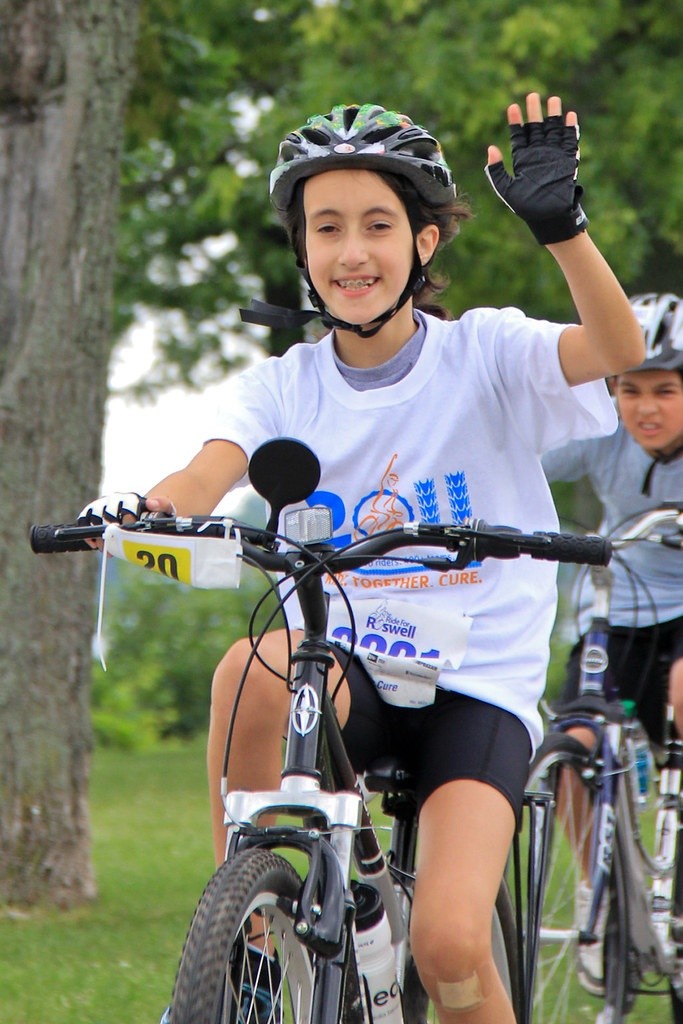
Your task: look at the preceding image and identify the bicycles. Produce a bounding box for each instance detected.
[25,435,619,1024]
[476,503,683,1024]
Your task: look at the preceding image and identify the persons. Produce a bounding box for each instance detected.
[77,90,644,1023]
[535,292,683,998]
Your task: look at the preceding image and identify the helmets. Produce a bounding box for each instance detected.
[270,103,457,245]
[628,292,683,369]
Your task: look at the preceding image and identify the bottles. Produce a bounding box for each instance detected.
[615,698,656,815]
[338,877,403,1024]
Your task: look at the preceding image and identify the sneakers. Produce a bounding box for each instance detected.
[158,944,286,1024]
[576,881,610,998]
